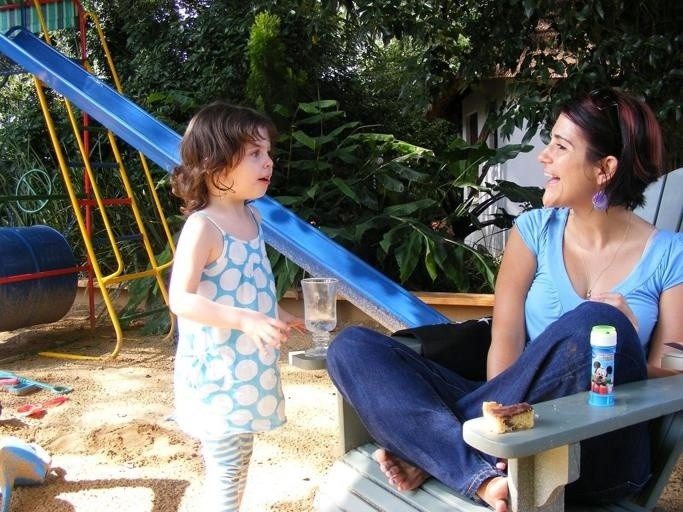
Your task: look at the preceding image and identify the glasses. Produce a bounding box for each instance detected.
[581,82,620,132]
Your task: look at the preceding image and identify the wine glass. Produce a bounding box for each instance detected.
[300,278,339,357]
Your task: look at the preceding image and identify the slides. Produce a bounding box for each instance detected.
[0,26,453,334]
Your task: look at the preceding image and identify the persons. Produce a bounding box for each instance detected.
[164,103,307,511]
[326,94,683,511]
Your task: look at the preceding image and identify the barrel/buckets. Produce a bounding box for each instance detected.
[0,224,77,332]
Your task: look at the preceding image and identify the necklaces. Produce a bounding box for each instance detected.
[573,211,633,296]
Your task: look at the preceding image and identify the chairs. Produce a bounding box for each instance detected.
[288,169,683,512]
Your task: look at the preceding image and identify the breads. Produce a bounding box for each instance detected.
[481,401,535,435]
